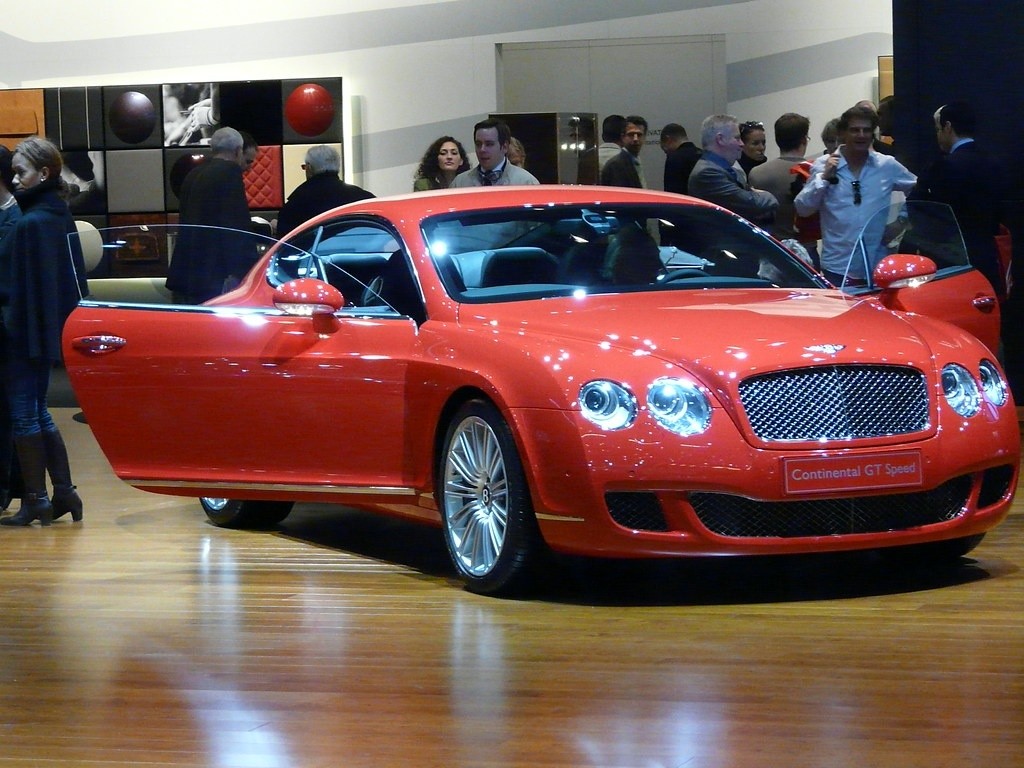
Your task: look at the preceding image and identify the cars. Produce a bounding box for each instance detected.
[61,185,1018,595]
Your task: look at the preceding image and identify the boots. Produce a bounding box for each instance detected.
[0,430,53,526]
[43,428,82,522]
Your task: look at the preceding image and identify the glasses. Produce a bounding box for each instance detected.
[740,120,763,134]
[806,135,811,142]
[851,179,862,205]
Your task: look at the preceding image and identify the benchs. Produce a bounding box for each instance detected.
[318,246,561,307]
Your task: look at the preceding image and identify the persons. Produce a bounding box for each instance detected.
[165,126,273,306]
[413,118,540,192]
[0,135,89,527]
[569,117,598,185]
[276,145,376,280]
[596,96,1007,297]
[187,82,221,132]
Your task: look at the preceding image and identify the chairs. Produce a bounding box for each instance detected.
[478,247,574,287]
[562,243,610,285]
[382,247,466,324]
